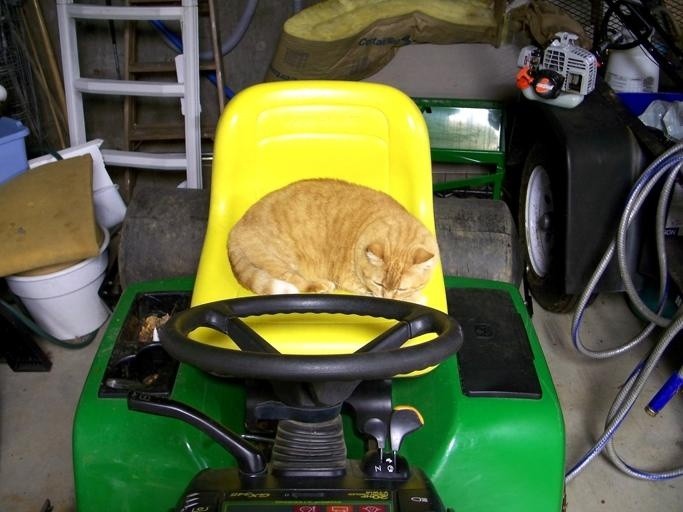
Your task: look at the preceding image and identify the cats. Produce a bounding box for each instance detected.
[225,176,437,307]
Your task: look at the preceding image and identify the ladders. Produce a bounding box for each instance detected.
[57,0,226,202]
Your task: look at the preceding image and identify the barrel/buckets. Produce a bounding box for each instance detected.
[4,224,111,341]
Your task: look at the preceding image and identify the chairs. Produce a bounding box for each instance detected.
[186,77,451,380]
